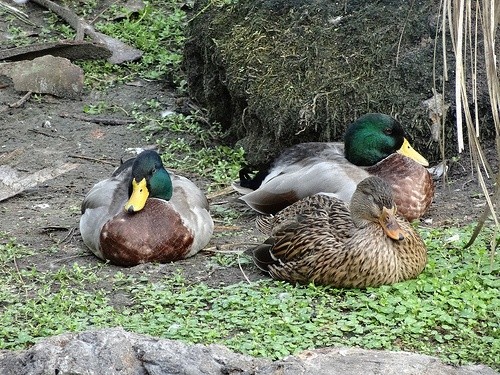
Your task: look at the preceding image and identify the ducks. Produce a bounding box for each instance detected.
[199,177,428,289]
[231,113,431,216]
[79,149,215,268]
